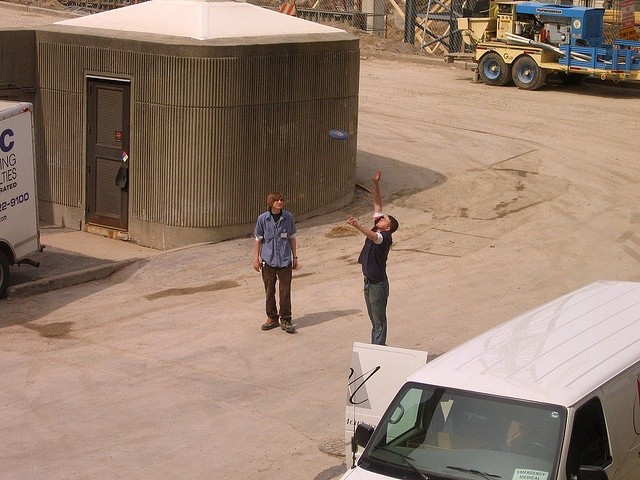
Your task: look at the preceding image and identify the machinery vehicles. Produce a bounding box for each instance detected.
[476,1,640,90]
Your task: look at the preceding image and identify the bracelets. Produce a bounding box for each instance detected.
[292,257,298,259]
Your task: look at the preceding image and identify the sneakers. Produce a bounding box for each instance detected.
[281,321,295,333]
[261,318,279,329]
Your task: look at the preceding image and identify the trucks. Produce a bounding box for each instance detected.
[340,280,640,480]
[0,100,41,299]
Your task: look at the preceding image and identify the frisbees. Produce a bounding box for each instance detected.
[328,129,349,140]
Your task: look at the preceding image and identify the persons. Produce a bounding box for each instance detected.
[347,170,399,346]
[253,191,298,334]
